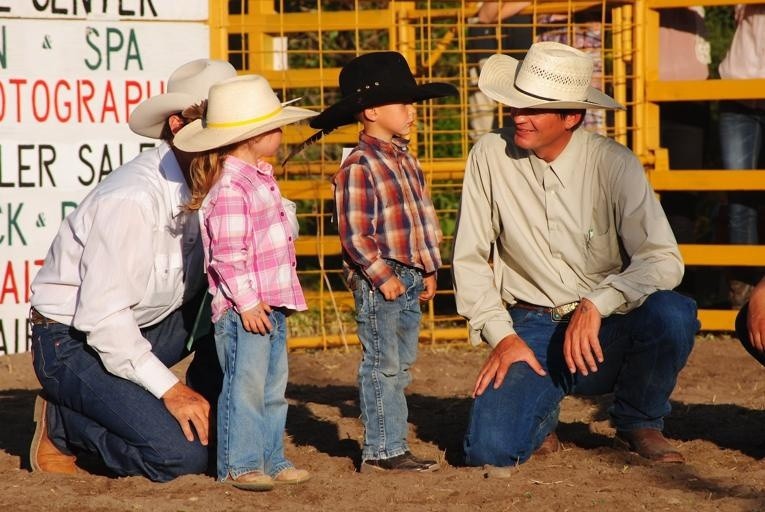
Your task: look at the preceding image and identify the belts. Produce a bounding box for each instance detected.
[29,310,57,325]
[516,299,583,320]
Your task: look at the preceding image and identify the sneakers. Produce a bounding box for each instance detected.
[275,467,312,484]
[226,473,275,491]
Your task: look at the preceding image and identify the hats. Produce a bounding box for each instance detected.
[127,58,238,139]
[477,40,626,110]
[171,74,321,153]
[309,50,458,128]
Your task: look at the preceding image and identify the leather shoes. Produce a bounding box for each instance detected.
[611,427,686,468]
[30,388,79,476]
[537,431,562,457]
[406,446,438,471]
[361,455,430,479]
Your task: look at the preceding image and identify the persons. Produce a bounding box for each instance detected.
[732,275,765,368]
[716,1,765,308]
[476,0,609,139]
[28,58,241,479]
[651,5,714,310]
[309,50,460,474]
[171,73,320,491]
[449,42,702,467]
[460,0,527,148]
